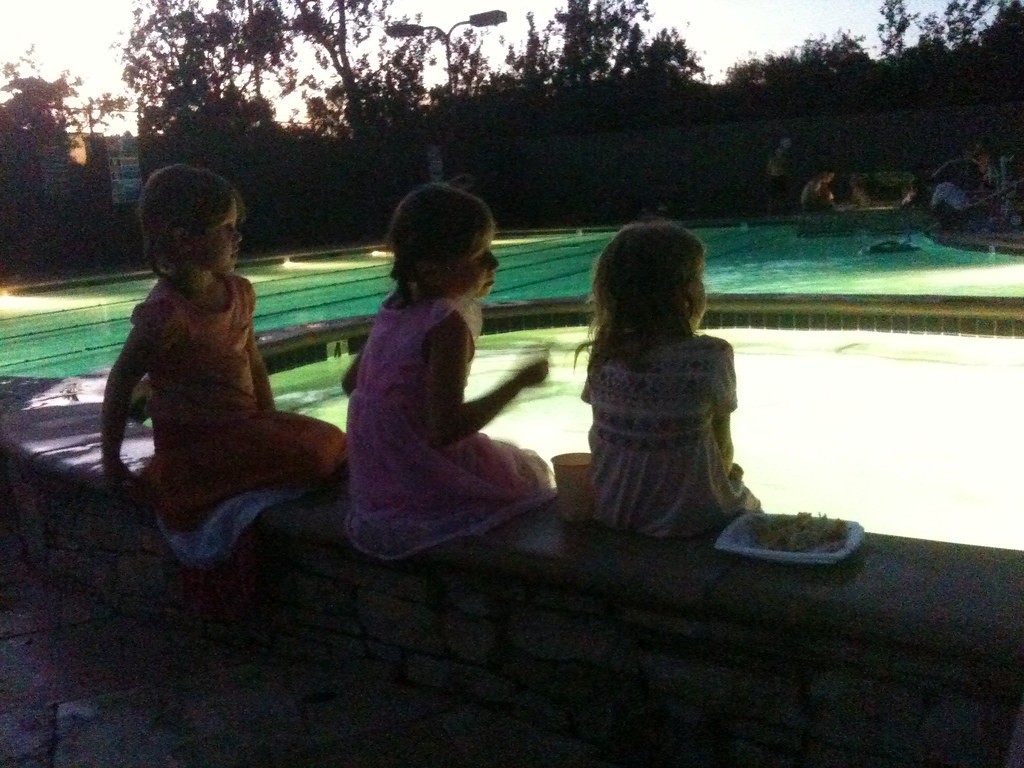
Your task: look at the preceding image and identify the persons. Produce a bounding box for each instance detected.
[91,162,348,517]
[797,163,973,226]
[341,181,561,561]
[579,221,768,538]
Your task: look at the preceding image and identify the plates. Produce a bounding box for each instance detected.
[715,513,865,565]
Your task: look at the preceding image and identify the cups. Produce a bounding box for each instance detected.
[553,454,596,523]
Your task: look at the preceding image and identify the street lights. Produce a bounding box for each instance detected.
[385,11,507,100]
